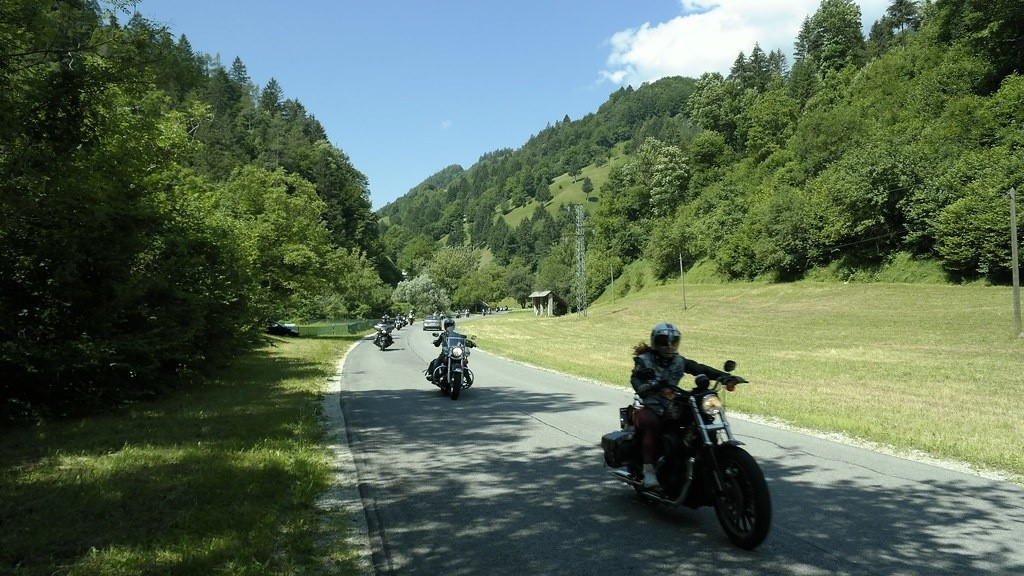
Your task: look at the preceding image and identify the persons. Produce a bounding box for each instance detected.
[630,323,746,487]
[431,305,508,318]
[425,319,476,385]
[372,309,415,343]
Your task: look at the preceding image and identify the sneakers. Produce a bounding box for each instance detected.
[642,463,660,488]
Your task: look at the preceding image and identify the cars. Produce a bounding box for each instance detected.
[265,320,300,338]
[422,315,442,331]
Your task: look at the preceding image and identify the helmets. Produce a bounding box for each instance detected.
[382,315,390,320]
[651,324,680,358]
[444,319,455,330]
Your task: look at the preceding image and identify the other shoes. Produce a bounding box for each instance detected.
[426,376,437,381]
[466,376,471,384]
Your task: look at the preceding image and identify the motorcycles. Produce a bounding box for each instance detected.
[602,361,771,551]
[372,324,394,351]
[381,315,415,330]
[432,307,507,320]
[422,332,478,400]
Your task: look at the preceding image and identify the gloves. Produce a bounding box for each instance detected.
[637,384,650,398]
[727,375,745,386]
